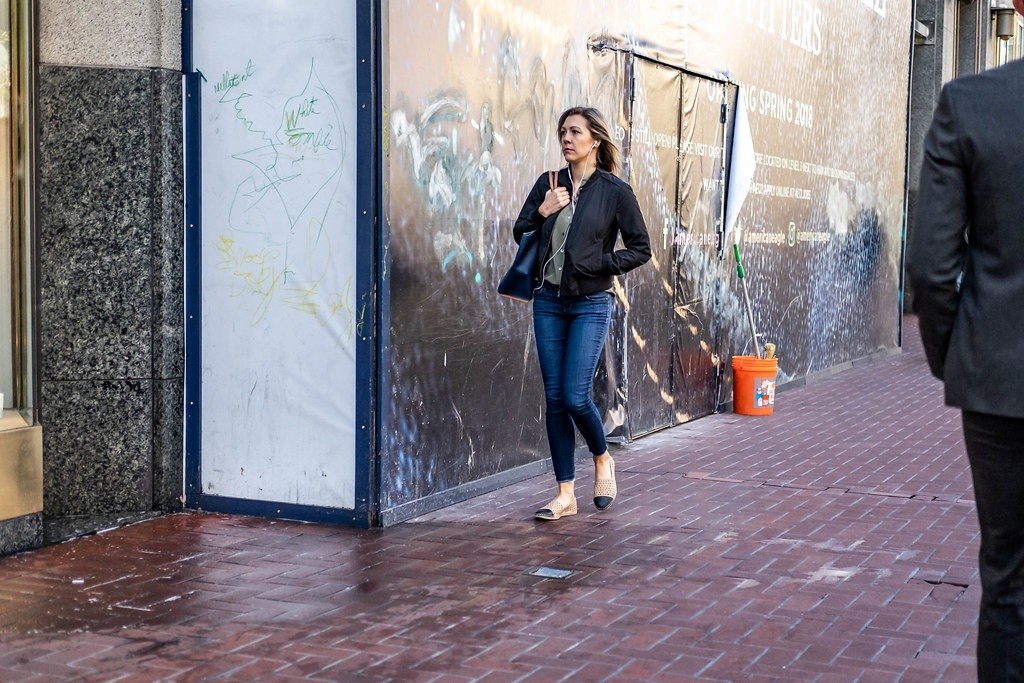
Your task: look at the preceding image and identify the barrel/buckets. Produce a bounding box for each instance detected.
[731,334,778,416]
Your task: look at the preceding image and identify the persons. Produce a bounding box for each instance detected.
[512,106,651,519]
[907,0,1024,682]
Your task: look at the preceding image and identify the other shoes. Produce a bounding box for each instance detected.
[594,455,617,511]
[534,497,577,520]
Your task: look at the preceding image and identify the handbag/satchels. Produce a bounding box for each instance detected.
[497,171,558,303]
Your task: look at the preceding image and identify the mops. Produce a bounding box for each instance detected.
[732,243,778,360]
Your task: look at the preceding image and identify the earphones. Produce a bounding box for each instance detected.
[592,139,598,148]
